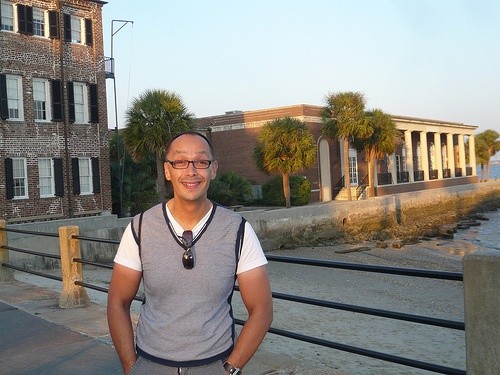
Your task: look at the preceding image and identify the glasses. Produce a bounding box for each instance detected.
[179,230,195,270]
[165,159,214,169]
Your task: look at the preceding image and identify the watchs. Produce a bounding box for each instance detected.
[222,360,242,375]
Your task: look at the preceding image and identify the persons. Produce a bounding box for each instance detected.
[106,131,273,375]
